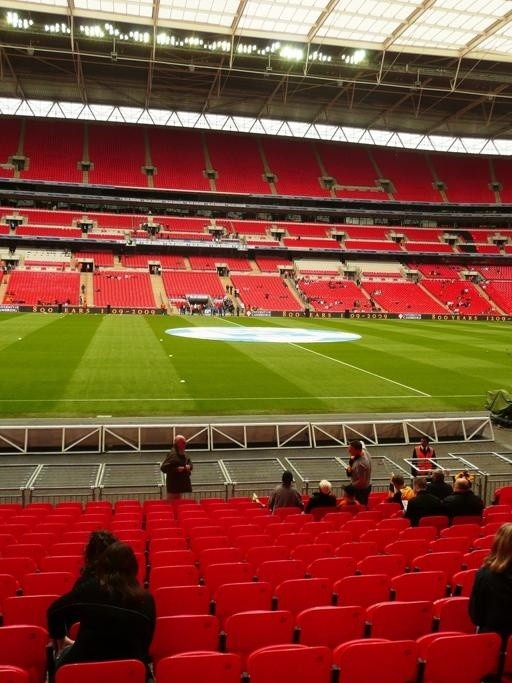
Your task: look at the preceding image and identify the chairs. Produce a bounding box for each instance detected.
[2,115,511,226]
[0,484,512,683]
[2,207,512,322]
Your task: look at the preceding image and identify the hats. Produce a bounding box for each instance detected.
[318,479,331,494]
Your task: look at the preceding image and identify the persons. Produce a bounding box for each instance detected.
[243,280,289,299]
[78,263,134,280]
[266,438,485,527]
[150,266,161,275]
[44,531,155,663]
[400,239,404,247]
[282,269,384,312]
[161,299,258,317]
[468,522,511,634]
[415,263,501,316]
[395,298,412,308]
[218,267,239,298]
[160,434,193,494]
[65,283,101,308]
